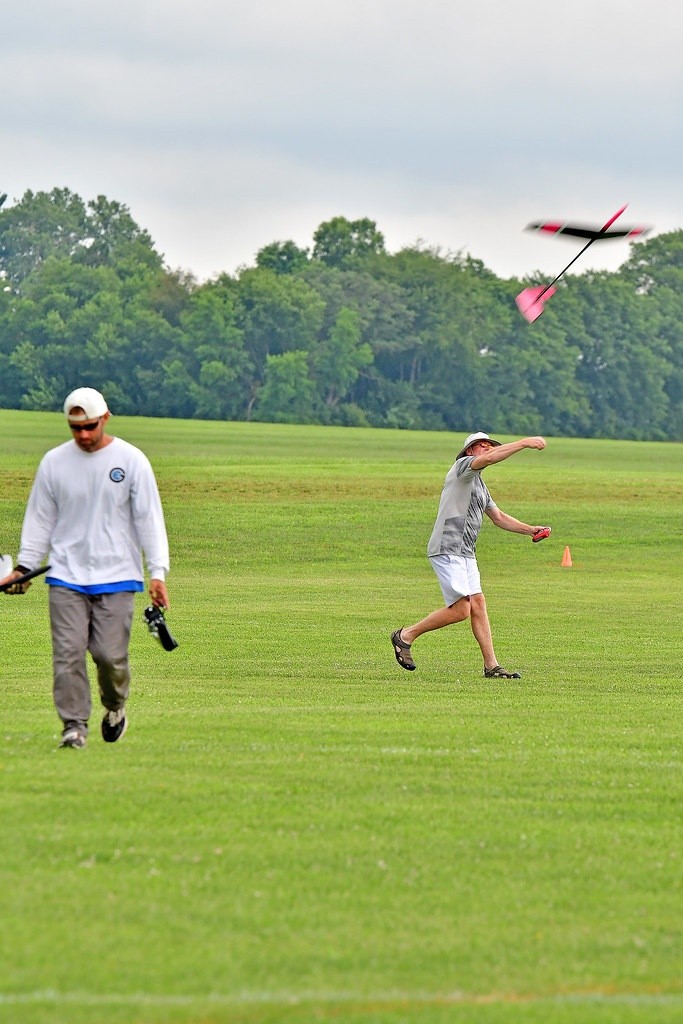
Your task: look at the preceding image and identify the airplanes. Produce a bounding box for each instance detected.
[514,203,654,324]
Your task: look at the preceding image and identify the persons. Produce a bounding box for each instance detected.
[0,386,170,749]
[391,430,551,679]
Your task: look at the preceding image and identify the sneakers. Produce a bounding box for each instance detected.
[101,706,128,743]
[58,721,88,749]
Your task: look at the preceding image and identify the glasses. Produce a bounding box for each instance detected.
[70,421,100,432]
[471,442,494,449]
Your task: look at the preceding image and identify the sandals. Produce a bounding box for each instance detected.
[391,627,417,671]
[484,665,521,679]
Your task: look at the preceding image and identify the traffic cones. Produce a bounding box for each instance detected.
[561,545,572,567]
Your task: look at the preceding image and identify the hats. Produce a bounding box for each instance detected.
[456,432,502,461]
[63,387,114,421]
